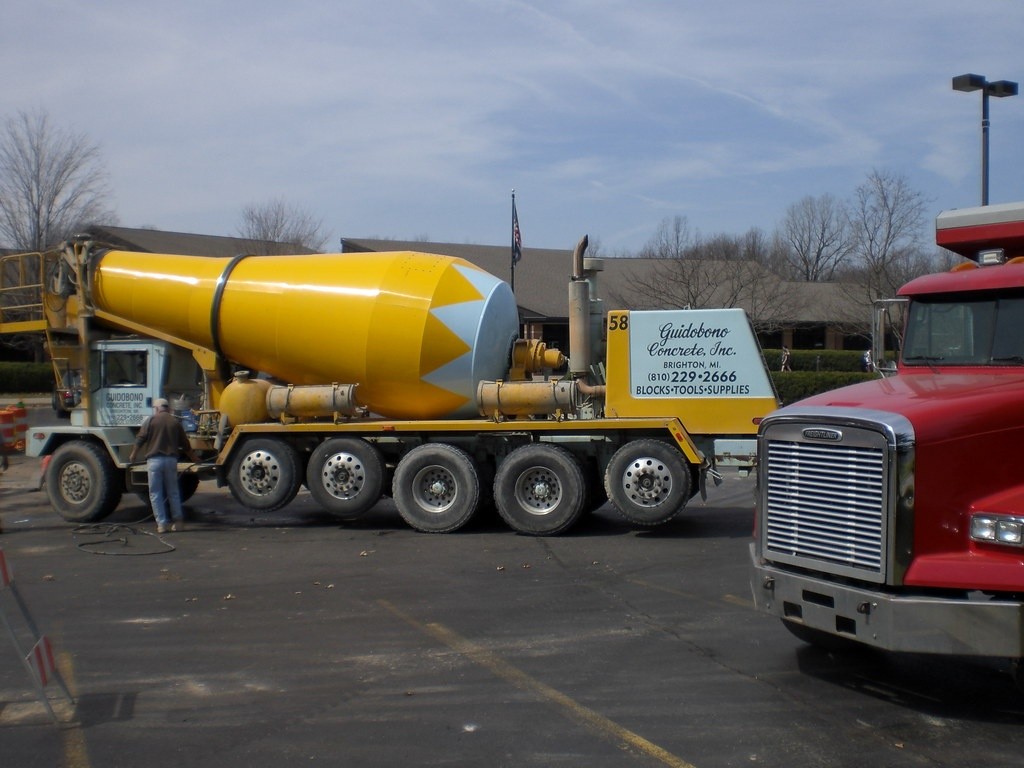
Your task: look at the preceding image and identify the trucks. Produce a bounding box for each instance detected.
[753,200,1024,661]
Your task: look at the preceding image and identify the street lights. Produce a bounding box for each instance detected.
[950,72,1019,208]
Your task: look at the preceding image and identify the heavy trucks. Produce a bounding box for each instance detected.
[0,235,785,538]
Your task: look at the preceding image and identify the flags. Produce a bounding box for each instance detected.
[514,205,522,264]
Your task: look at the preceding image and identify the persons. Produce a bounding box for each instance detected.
[780,345,792,372]
[128,398,201,532]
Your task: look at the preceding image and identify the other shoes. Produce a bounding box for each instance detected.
[171,521,184,531]
[157,526,167,533]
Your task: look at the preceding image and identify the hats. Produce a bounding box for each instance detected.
[153,398,169,408]
